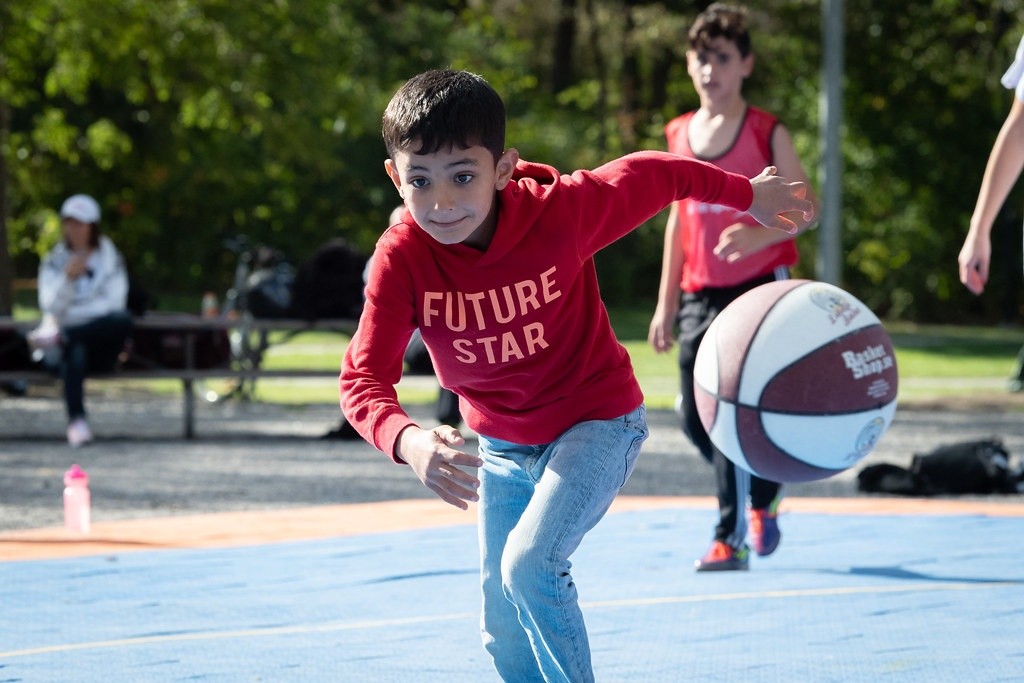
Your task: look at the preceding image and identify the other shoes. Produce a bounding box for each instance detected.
[27,328,60,353]
[66,419,92,449]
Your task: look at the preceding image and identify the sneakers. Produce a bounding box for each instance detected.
[697,539,750,574]
[750,499,782,555]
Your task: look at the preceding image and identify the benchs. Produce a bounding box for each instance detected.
[1,311,234,440]
[237,315,473,429]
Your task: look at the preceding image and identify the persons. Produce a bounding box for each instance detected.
[958,34,1024,294]
[648,3,820,572]
[339,69,815,683]
[27,194,131,446]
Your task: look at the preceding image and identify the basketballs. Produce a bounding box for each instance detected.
[692,279,900,484]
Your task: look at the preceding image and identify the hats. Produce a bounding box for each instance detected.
[61,193,103,224]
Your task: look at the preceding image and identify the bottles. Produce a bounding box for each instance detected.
[62,464,91,533]
[202,292,217,321]
